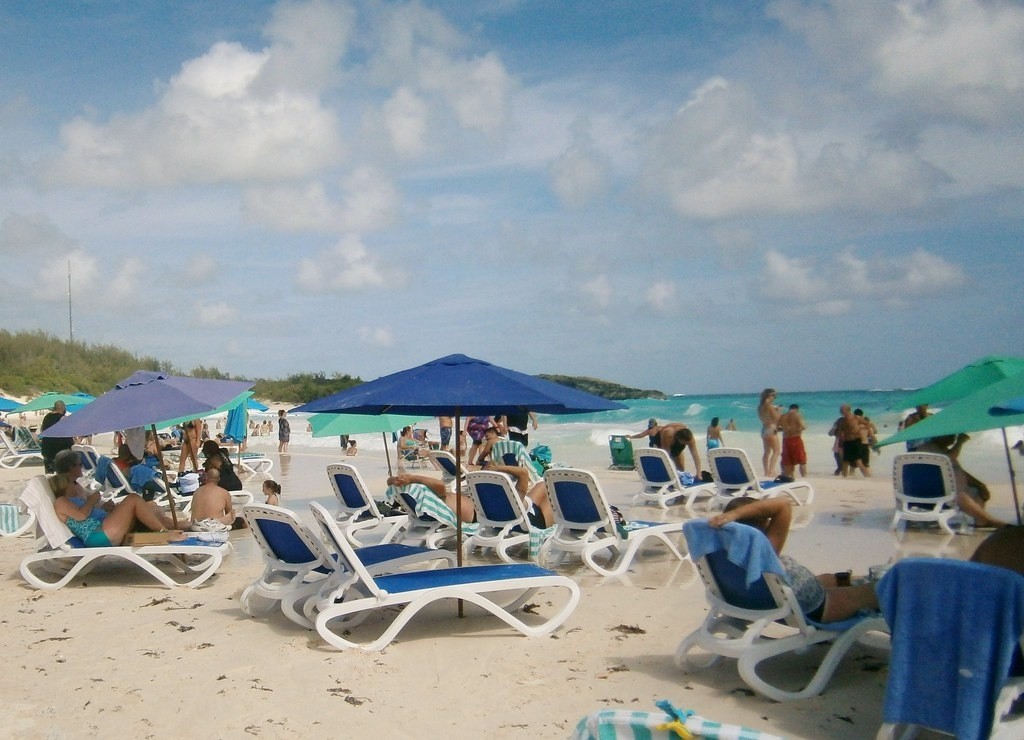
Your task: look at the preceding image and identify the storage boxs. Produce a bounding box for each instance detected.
[123,533,168,546]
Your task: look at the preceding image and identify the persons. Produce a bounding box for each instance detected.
[727,418,737,431]
[828,404,881,477]
[706,416,725,451]
[624,418,703,480]
[340,410,555,533]
[1,400,282,547]
[758,389,809,478]
[898,402,1012,528]
[707,497,880,622]
[278,411,290,453]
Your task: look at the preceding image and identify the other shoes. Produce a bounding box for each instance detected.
[197,532,229,542]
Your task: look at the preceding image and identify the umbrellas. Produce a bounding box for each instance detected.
[63,392,98,414]
[10,391,96,414]
[247,397,270,413]
[871,367,1023,449]
[0,397,26,412]
[309,413,436,477]
[223,400,246,474]
[287,352,629,617]
[144,391,254,472]
[887,354,1024,525]
[38,370,256,575]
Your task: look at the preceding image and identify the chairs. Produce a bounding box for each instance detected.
[0,421,1023,740]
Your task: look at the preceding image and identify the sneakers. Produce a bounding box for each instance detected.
[191,518,232,532]
